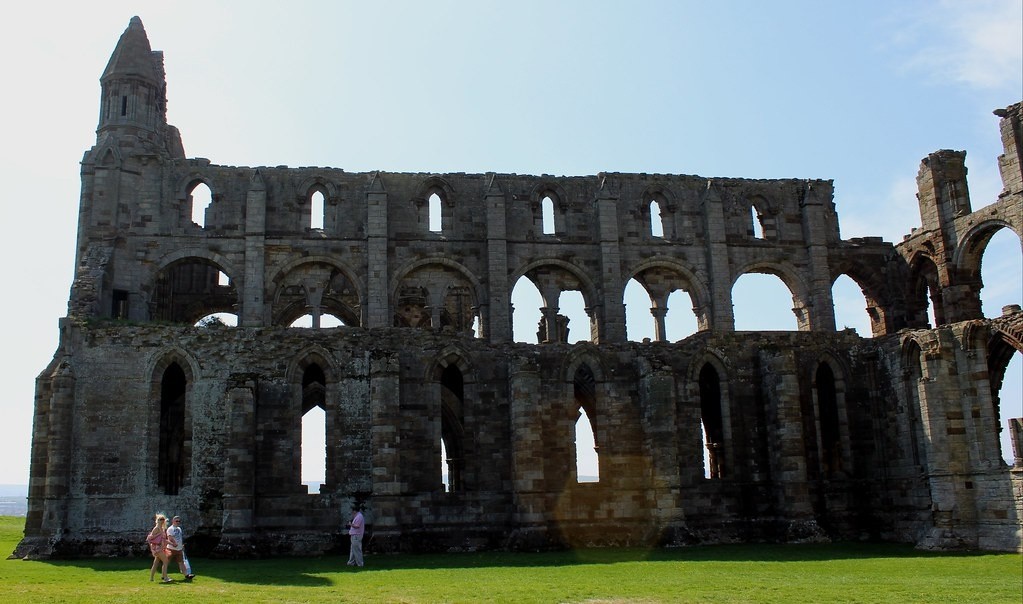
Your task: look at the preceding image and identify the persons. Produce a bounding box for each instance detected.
[346,505,365,567]
[145,514,171,582]
[160,515,195,580]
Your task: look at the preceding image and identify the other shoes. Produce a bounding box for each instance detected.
[161,574,195,583]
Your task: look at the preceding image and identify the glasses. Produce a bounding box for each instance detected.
[158,519,165,521]
[175,520,181,522]
